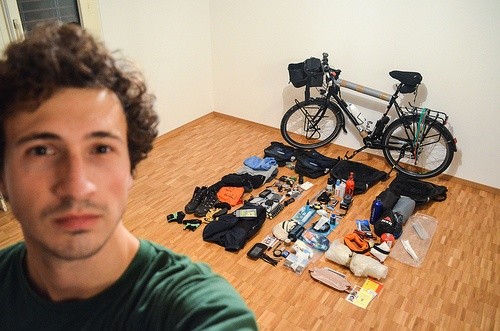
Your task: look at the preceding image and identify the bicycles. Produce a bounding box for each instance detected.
[281,52,458,180]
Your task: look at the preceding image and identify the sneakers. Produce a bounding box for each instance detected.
[185,185,207,213]
[194,187,218,217]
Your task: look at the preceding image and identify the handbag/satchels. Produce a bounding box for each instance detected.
[264,142,301,167]
[295,148,339,179]
[329,159,389,195]
[287,57,323,89]
[389,172,447,205]
[373,207,404,238]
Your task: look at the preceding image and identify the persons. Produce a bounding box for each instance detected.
[0,20,258,331]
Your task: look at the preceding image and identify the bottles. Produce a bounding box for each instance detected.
[370,197,383,224]
[327,172,355,198]
[349,104,365,124]
